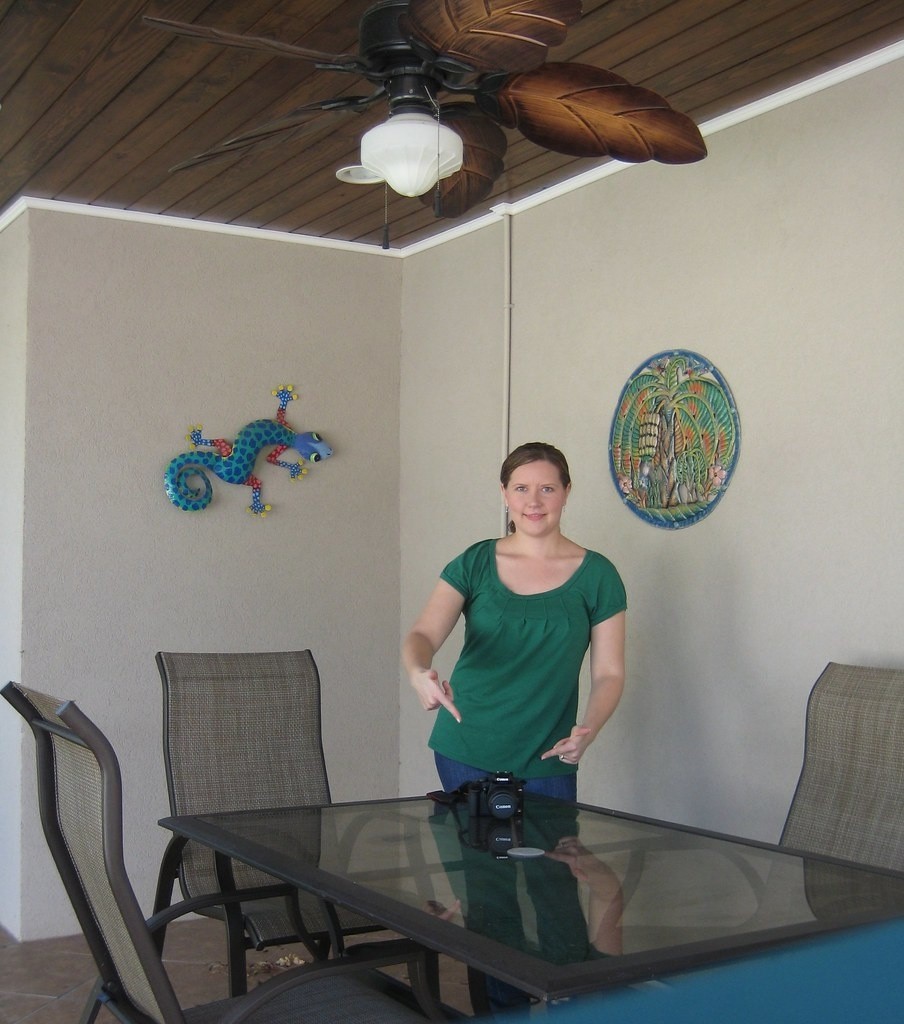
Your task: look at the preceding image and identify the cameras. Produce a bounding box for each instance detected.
[469,818,526,860]
[469,769,526,820]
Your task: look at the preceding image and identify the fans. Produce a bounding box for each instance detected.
[141,0,709,221]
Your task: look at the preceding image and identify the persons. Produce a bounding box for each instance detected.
[421,798,624,966]
[403,442,628,1024]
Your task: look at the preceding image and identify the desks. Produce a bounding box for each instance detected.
[159,790,904,1024]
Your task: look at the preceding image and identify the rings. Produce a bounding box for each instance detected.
[431,912,436,916]
[560,755,564,759]
[561,840,569,848]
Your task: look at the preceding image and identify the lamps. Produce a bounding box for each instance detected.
[360,114,463,250]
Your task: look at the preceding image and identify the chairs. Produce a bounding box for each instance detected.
[0,679,474,1024]
[146,648,442,1024]
[778,661,904,875]
